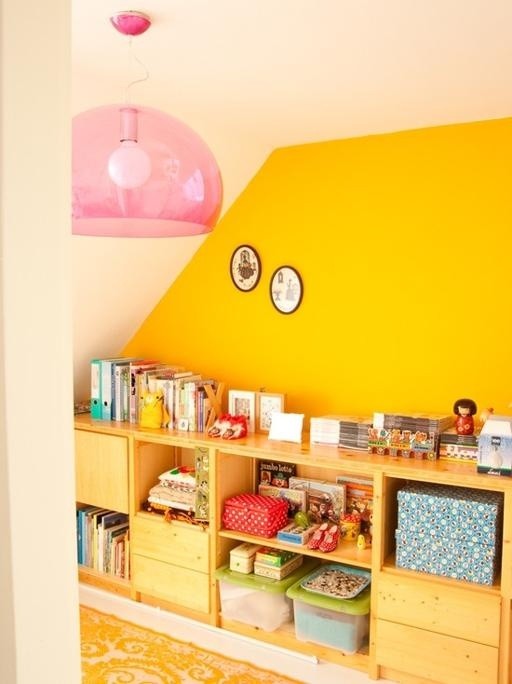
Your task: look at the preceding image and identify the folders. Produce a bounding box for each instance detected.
[91,357,145,421]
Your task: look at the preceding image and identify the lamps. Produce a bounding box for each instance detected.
[70,11,223,238]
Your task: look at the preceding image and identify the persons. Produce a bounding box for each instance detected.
[454,399,477,436]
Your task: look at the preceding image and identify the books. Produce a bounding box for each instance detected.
[89,358,216,433]
[309,409,479,461]
[257,459,375,526]
[76,506,130,582]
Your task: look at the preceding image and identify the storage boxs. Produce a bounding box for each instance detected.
[394,529,501,586]
[213,557,325,633]
[397,481,504,545]
[285,561,370,657]
[476,413,512,477]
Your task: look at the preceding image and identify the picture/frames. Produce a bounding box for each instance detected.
[230,245,261,292]
[227,388,257,434]
[269,265,304,314]
[257,391,287,435]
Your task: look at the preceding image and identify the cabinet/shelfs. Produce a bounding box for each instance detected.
[74,412,512,684]
